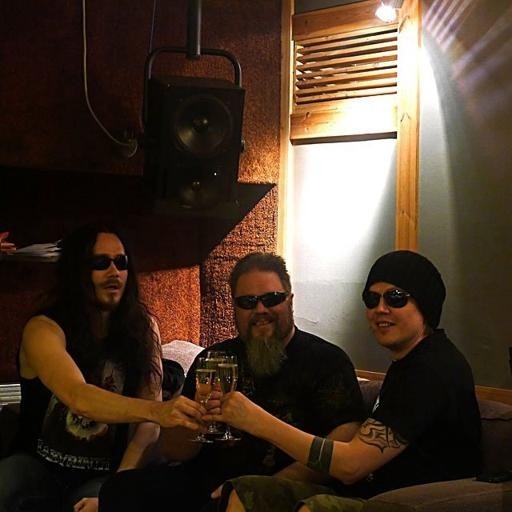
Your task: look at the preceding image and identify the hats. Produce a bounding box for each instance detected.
[361,249,447,329]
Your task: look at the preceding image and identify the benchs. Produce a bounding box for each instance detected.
[162,340,512,511]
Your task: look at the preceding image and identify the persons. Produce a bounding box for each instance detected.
[99,252,361,512]
[19,223,206,512]
[203,250,483,512]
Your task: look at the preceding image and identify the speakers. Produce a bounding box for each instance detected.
[143,75,245,219]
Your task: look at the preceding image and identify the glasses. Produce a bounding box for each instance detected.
[361,287,413,310]
[91,253,129,271]
[233,290,289,310]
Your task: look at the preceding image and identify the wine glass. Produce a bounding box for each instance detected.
[188,350,243,445]
